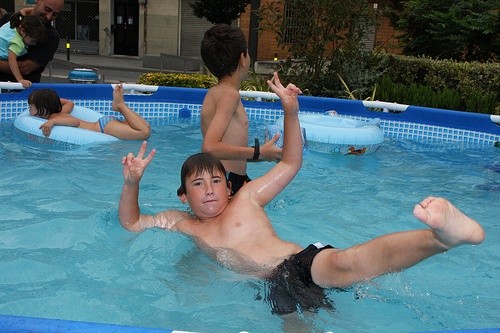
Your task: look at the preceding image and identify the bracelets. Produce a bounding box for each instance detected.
[251,138,260,160]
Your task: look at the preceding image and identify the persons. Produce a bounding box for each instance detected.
[201,23,283,195]
[118,72,485,333]
[0,0,64,88]
[27,83,151,140]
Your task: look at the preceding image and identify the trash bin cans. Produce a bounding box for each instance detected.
[66,68,98,84]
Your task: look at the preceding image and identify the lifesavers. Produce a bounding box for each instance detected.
[270,112,386,156]
[13,103,121,147]
